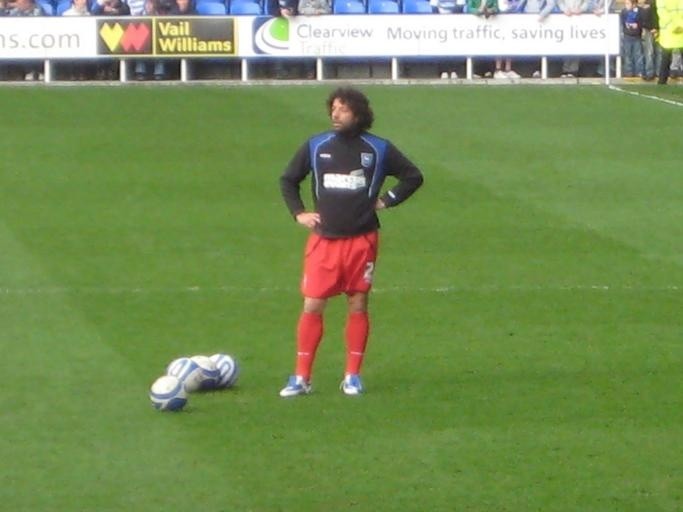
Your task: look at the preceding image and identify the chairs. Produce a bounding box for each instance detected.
[30,0,467,17]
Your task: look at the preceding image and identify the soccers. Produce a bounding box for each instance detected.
[168,353,238,394]
[149,377,189,413]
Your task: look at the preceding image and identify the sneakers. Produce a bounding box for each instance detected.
[560,72,578,78]
[68,67,117,80]
[24,71,44,81]
[135,73,163,81]
[623,72,678,83]
[279,374,312,396]
[342,373,362,394]
[271,69,316,80]
[440,71,458,78]
[473,70,521,79]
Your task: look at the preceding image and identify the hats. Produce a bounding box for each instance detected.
[278,0,298,9]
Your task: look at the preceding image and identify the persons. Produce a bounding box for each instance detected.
[274,84,425,400]
